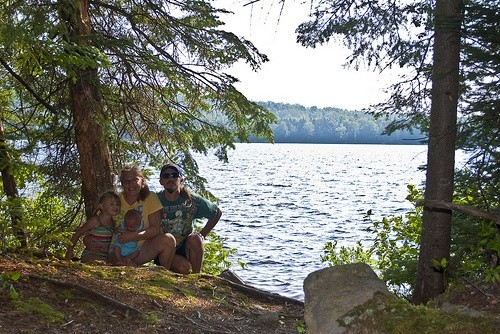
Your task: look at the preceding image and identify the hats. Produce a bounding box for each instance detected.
[161,164,180,174]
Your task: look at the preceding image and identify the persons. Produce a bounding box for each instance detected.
[108,165,177,271]
[156,164,222,273]
[65,192,121,263]
[114,210,144,267]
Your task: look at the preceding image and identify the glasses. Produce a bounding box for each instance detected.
[161,172,179,179]
